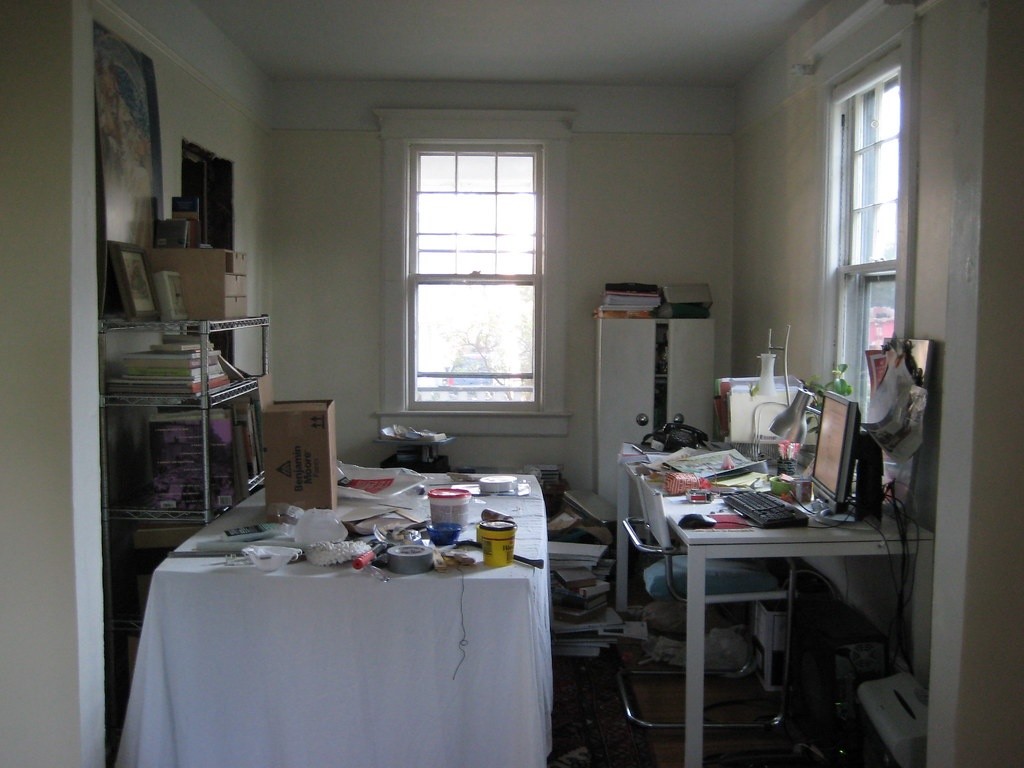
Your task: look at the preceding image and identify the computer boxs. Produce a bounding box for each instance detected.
[782,598,888,768]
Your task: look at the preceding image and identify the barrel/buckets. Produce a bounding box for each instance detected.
[479,519,518,568]
[427,488,471,534]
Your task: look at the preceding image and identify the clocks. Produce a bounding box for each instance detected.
[152,269,189,322]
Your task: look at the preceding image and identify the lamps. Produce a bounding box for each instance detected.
[769,389,823,446]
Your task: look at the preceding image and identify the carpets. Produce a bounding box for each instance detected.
[552,649,658,768]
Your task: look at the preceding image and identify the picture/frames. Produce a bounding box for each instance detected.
[107,240,159,323]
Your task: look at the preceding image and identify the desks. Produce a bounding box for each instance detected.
[112,474,554,768]
[615,443,933,768]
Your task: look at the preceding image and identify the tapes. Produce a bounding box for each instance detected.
[387,544,433,575]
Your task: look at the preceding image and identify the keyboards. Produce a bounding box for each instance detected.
[724,491,809,530]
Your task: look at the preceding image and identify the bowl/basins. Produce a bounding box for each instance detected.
[426,523,462,546]
[769,476,793,496]
[243,546,294,572]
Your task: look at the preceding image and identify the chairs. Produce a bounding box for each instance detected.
[615,476,799,730]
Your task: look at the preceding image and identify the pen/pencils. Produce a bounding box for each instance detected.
[779,441,799,460]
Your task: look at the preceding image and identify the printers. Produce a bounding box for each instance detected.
[841,672,928,768]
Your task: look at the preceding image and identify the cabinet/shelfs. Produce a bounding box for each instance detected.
[595,317,717,508]
[98,314,271,761]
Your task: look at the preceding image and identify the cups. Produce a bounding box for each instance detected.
[795,481,812,503]
[778,460,796,477]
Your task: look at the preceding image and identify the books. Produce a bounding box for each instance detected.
[602,282,660,312]
[548,542,647,657]
[156,196,199,249]
[716,376,807,442]
[150,401,262,509]
[663,449,759,479]
[108,335,229,394]
[518,464,559,484]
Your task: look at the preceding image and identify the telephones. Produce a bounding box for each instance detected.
[663,422,709,453]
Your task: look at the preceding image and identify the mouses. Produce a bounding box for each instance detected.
[678,513,717,530]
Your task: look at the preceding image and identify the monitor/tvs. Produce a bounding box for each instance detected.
[813,389,886,531]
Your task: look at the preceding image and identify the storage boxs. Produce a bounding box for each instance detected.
[130,524,201,611]
[145,247,249,321]
[749,599,788,692]
[256,373,337,522]
